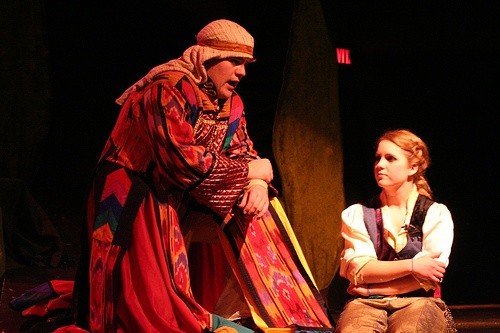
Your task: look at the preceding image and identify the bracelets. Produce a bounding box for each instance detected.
[248,179,269,190]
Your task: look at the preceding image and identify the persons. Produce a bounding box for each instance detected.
[333,130,458,333]
[70,18,273,333]
